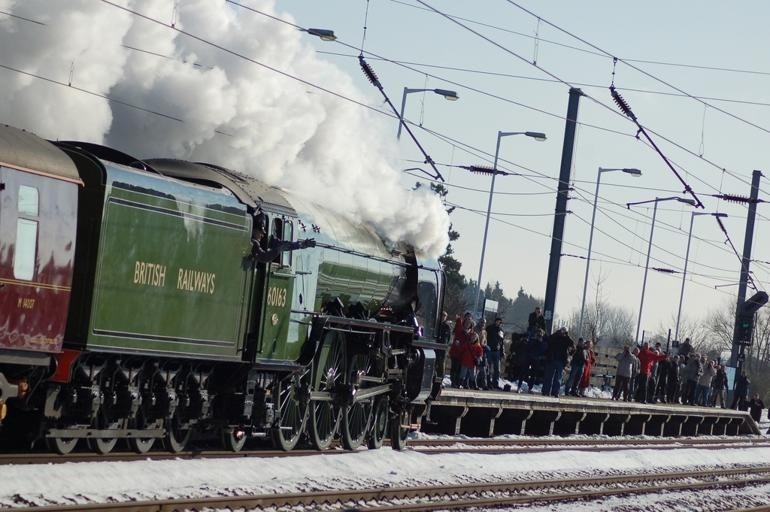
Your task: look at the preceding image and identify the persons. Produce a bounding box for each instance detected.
[611,338,729,408]
[730,371,766,423]
[436,311,505,391]
[251,212,316,264]
[504,308,595,399]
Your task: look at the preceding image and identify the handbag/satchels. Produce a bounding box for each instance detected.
[474,356,482,366]
[484,344,491,352]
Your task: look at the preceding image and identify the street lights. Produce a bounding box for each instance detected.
[473,130,547,316]
[577,167,642,337]
[635,197,695,344]
[675,212,728,341]
[398,88,459,140]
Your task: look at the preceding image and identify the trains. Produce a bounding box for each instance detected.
[0,121,446,455]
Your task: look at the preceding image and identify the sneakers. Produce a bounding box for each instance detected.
[515,389,586,398]
[612,396,725,408]
[451,384,502,391]
